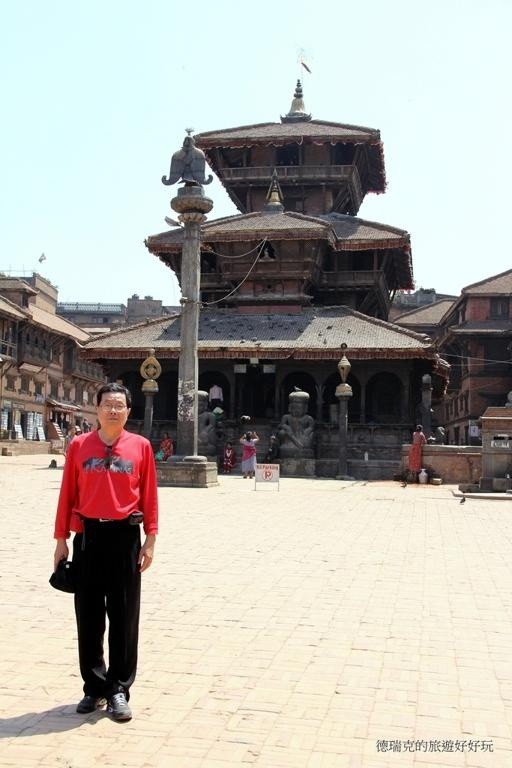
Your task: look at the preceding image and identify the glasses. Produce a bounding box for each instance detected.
[105,446,112,469]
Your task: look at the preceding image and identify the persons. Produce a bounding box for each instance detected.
[54,382,159,722]
[240,431,260,479]
[209,382,223,412]
[263,435,280,464]
[408,425,427,483]
[223,442,237,473]
[155,432,174,461]
[188,389,218,449]
[280,391,317,446]
[62,417,101,457]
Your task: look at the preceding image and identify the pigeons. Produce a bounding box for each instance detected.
[460,496,466,504]
[197,315,334,348]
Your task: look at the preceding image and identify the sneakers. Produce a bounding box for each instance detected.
[76,693,132,720]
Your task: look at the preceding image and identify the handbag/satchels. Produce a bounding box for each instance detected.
[49,560,75,593]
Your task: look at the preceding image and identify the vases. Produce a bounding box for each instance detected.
[418,468,428,484]
